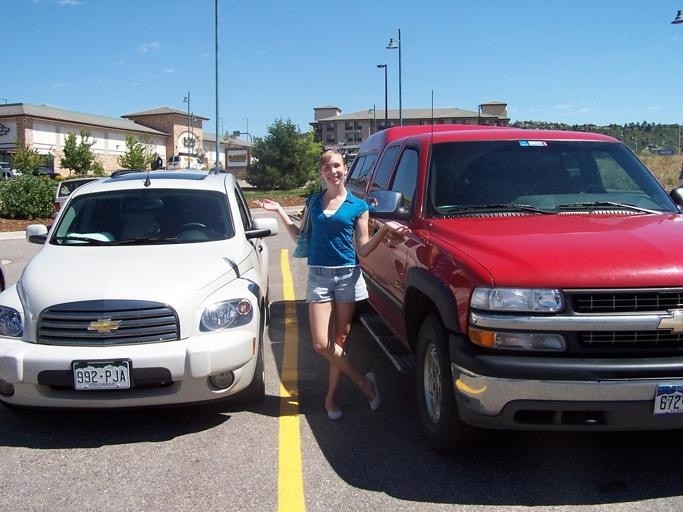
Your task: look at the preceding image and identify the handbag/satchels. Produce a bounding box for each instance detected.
[293,192,320,258]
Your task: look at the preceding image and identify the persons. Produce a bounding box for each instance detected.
[251,148,413,420]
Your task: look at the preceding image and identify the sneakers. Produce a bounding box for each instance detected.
[328,406,343,420]
[365,371,381,411]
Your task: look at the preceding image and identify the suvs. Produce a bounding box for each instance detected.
[342,124,683,448]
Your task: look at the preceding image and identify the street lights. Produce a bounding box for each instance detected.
[219,116,223,136]
[368,28,402,134]
[182,91,190,168]
[241,117,248,141]
